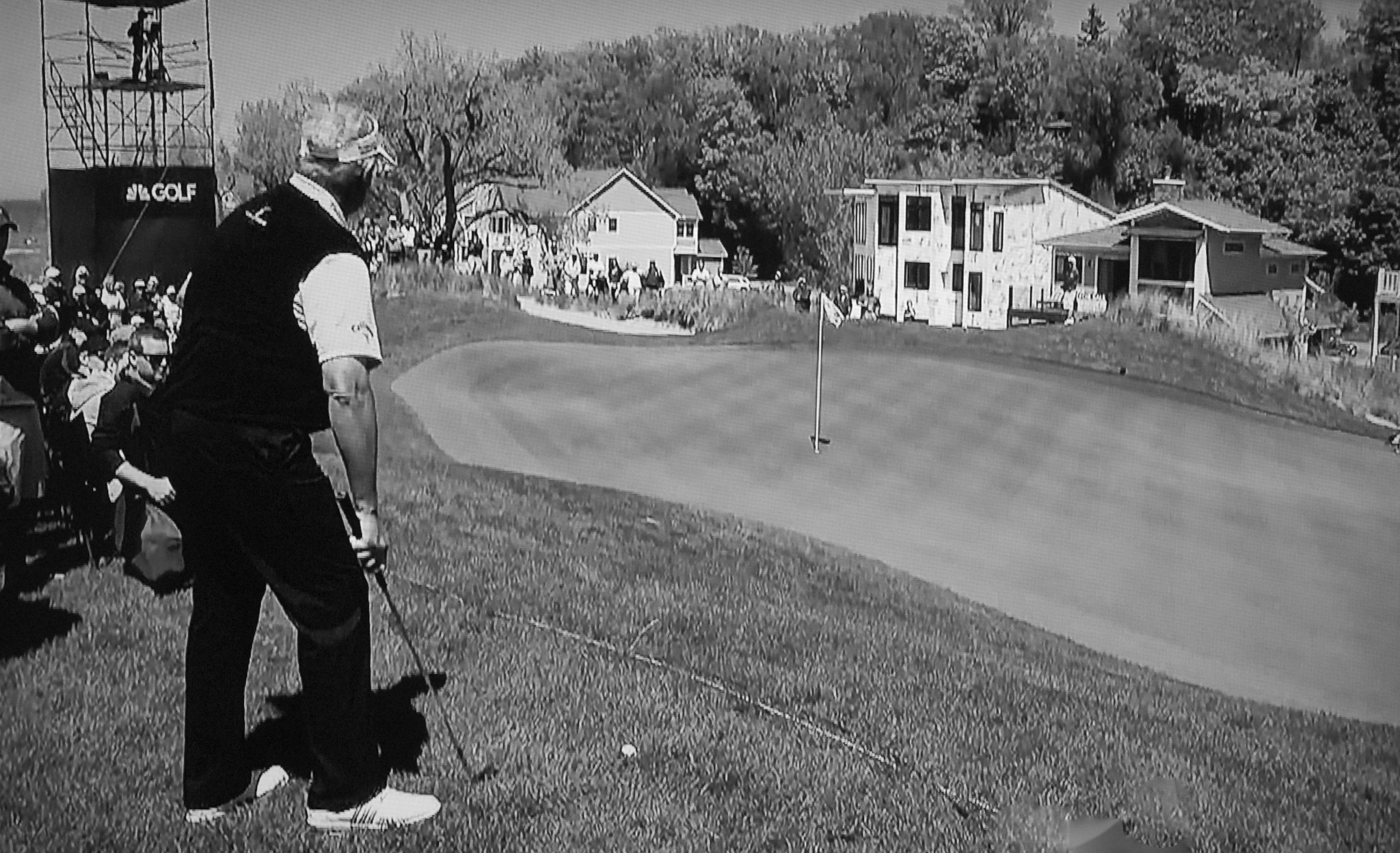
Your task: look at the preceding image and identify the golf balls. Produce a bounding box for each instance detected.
[622,744,637,758]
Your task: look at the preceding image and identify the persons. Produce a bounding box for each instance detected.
[1309,321,1324,360]
[1059,255,1079,325]
[128,7,155,80]
[93,326,198,563]
[342,208,923,333]
[150,102,444,833]
[0,204,63,599]
[23,255,185,529]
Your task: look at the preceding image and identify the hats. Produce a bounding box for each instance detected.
[299,104,398,167]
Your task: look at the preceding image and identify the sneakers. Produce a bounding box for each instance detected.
[303,783,442,830]
[184,764,290,824]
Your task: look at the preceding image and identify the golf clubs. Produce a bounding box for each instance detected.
[336,490,494,784]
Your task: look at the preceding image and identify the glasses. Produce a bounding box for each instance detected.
[133,350,173,365]
